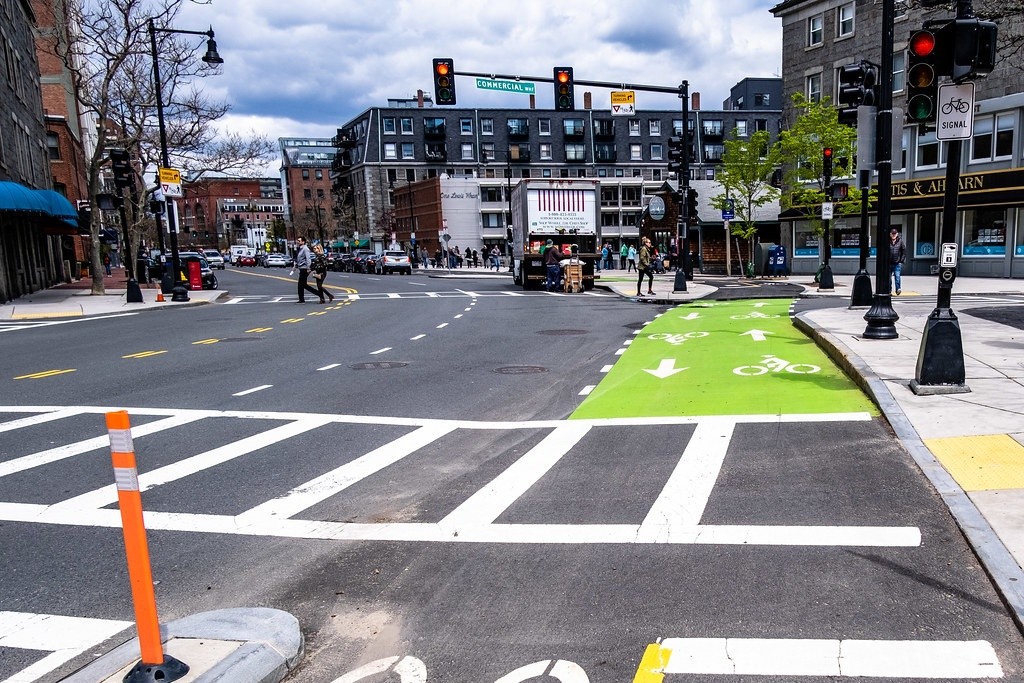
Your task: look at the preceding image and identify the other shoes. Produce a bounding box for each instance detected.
[329,295,334,303]
[318,291,325,300]
[637,292,646,297]
[648,291,656,295]
[296,300,306,304]
[896,289,901,295]
[318,300,325,304]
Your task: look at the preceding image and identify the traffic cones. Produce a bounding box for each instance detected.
[155,285,166,302]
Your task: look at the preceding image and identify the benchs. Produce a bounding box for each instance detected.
[467,259,505,267]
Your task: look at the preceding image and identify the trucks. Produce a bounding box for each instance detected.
[504,178,603,291]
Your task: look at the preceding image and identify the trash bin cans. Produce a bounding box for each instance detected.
[768,245,787,278]
[754,243,775,278]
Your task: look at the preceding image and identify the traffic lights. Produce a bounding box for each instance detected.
[150,199,166,215]
[833,182,848,199]
[95,193,118,211]
[823,147,833,176]
[904,28,949,125]
[688,188,699,217]
[938,18,979,80]
[432,57,456,106]
[553,66,575,113]
[110,149,131,188]
[836,63,866,127]
[667,135,682,173]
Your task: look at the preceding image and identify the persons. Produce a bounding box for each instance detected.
[889,228,906,295]
[637,236,678,297]
[290,237,320,304]
[435,248,441,268]
[472,248,479,268]
[596,244,615,273]
[307,244,334,304]
[465,246,472,268]
[480,245,489,269]
[430,257,437,268]
[490,245,501,271]
[103,253,112,277]
[543,239,564,292]
[421,247,429,269]
[198,248,207,267]
[619,242,638,274]
[447,246,461,268]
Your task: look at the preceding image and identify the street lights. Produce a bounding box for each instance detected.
[147,17,225,303]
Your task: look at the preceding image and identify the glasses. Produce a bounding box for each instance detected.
[647,241,651,243]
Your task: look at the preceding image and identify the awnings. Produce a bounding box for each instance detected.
[350,239,369,246]
[333,242,344,247]
[0,180,52,213]
[33,190,79,220]
[61,217,78,229]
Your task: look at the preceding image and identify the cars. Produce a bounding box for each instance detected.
[163,242,415,290]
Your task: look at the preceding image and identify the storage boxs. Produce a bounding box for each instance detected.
[530,241,571,255]
[564,265,582,292]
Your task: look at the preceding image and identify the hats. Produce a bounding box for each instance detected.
[890,227,899,234]
[547,239,553,244]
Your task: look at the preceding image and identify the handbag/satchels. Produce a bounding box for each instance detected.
[312,273,321,279]
